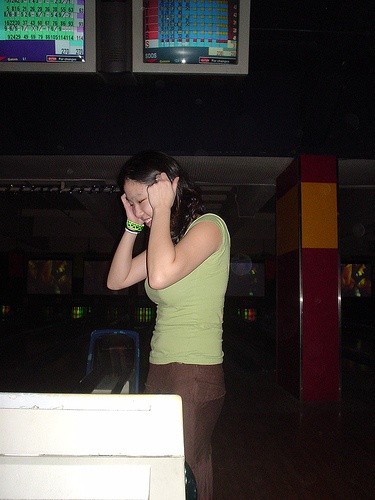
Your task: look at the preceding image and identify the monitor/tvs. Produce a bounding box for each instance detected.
[0,0,96,72]
[132,0,250,76]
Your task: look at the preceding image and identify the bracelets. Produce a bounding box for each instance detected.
[125,220,145,235]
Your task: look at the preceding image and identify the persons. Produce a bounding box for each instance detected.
[106,150,231,500]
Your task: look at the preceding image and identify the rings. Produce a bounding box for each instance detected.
[154,177,161,182]
